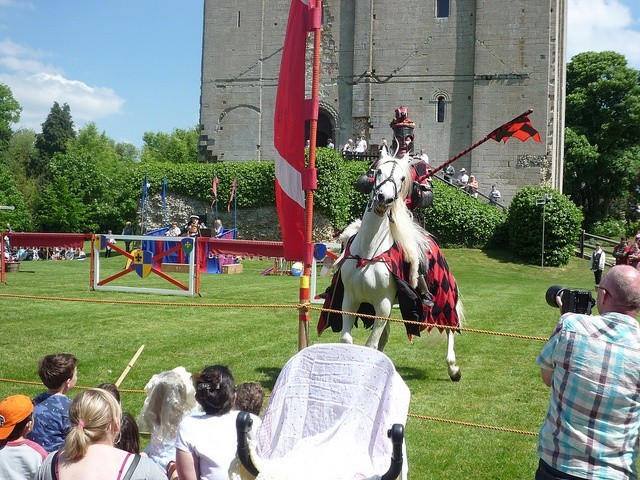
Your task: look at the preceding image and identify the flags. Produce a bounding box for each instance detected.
[138,174,147,208]
[227,178,236,212]
[273,0,308,261]
[161,176,167,208]
[489,115,544,145]
[210,176,218,213]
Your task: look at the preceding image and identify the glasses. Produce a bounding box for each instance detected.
[595,285,612,298]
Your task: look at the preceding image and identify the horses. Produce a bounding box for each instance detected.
[339,137,465,383]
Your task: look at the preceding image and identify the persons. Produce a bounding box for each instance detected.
[34,388,168,480]
[589,242,606,285]
[165,222,181,237]
[113,411,140,455]
[416,149,430,165]
[467,174,479,199]
[174,363,262,480]
[27,352,79,452]
[488,184,501,206]
[353,106,435,308]
[121,221,133,253]
[353,135,368,153]
[628,241,640,268]
[214,219,224,238]
[379,138,388,152]
[232,381,265,417]
[611,237,631,265]
[96,383,121,407]
[0,394,48,479]
[304,137,353,154]
[186,214,202,238]
[456,167,469,189]
[533,264,639,480]
[1,220,86,261]
[105,230,116,258]
[442,160,455,184]
[144,371,191,468]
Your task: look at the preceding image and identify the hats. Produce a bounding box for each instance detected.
[0,394,35,440]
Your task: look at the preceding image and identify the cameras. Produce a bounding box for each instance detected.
[545,285,596,314]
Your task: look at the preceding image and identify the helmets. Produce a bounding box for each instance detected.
[389,106,415,129]
